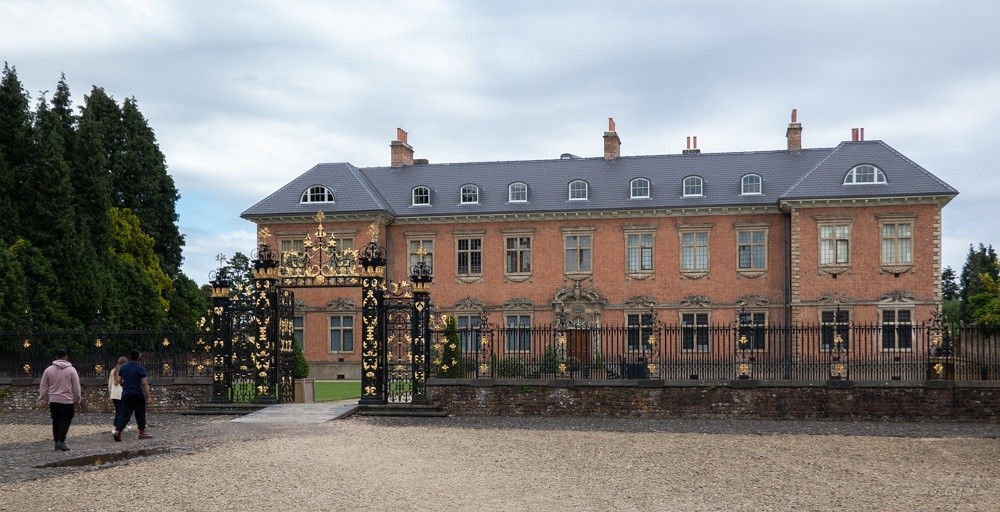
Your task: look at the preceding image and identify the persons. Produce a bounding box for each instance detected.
[39,349,83,451]
[108,356,128,434]
[112,350,153,442]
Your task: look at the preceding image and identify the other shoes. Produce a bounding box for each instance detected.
[54,440,71,451]
[113,431,122,442]
[139,430,153,439]
[112,426,116,434]
